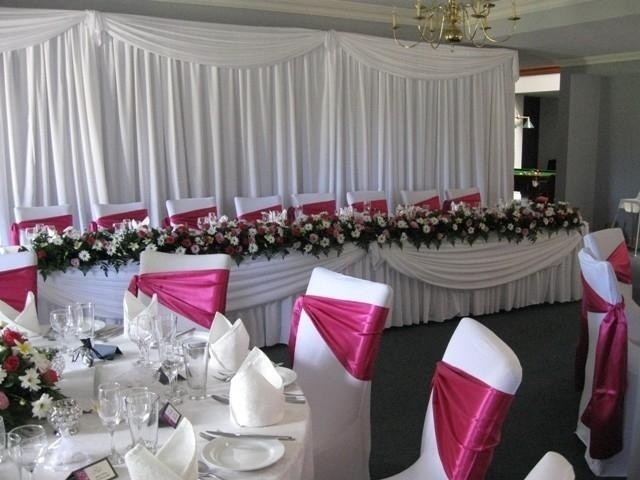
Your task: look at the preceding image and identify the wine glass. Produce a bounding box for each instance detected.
[6,423,48,480]
[93,382,124,461]
[49,397,85,460]
[127,313,182,404]
[47,307,71,355]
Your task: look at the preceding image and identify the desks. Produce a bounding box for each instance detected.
[514,168,556,203]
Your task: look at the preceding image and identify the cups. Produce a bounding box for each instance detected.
[68,300,96,352]
[117,387,160,455]
[180,338,210,401]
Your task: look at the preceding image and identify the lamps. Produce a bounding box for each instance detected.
[516,114,534,129]
[390,0,521,52]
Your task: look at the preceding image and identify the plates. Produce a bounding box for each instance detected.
[198,437,289,475]
[224,362,300,390]
[173,330,210,347]
